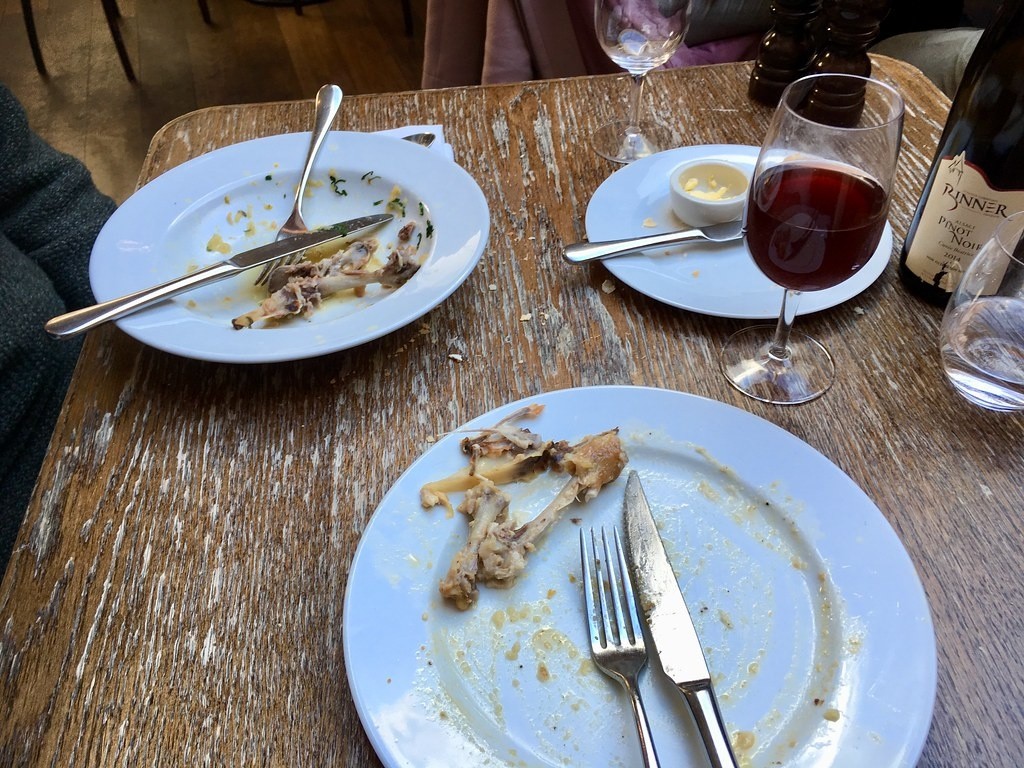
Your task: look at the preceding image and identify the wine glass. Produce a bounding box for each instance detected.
[719,73,903,404]
[592,0,692,162]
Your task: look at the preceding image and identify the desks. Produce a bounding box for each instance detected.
[1,54,1024,768]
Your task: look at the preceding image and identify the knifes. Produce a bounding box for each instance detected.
[43,213,394,342]
[561,219,746,265]
[624,471,736,767]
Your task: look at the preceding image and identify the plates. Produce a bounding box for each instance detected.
[89,132,489,362]
[585,144,893,320]
[344,384,936,767]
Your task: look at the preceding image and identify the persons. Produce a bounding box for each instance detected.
[868,25,985,101]
[0,84,120,583]
[419,0,765,89]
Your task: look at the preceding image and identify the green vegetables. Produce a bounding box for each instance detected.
[264,171,435,251]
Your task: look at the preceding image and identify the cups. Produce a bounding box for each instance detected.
[938,211,1024,412]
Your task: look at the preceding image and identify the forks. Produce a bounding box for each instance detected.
[254,85,342,291]
[578,530,662,767]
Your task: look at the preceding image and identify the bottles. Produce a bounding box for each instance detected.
[898,0,1023,306]
[750,0,823,108]
[795,0,891,127]
